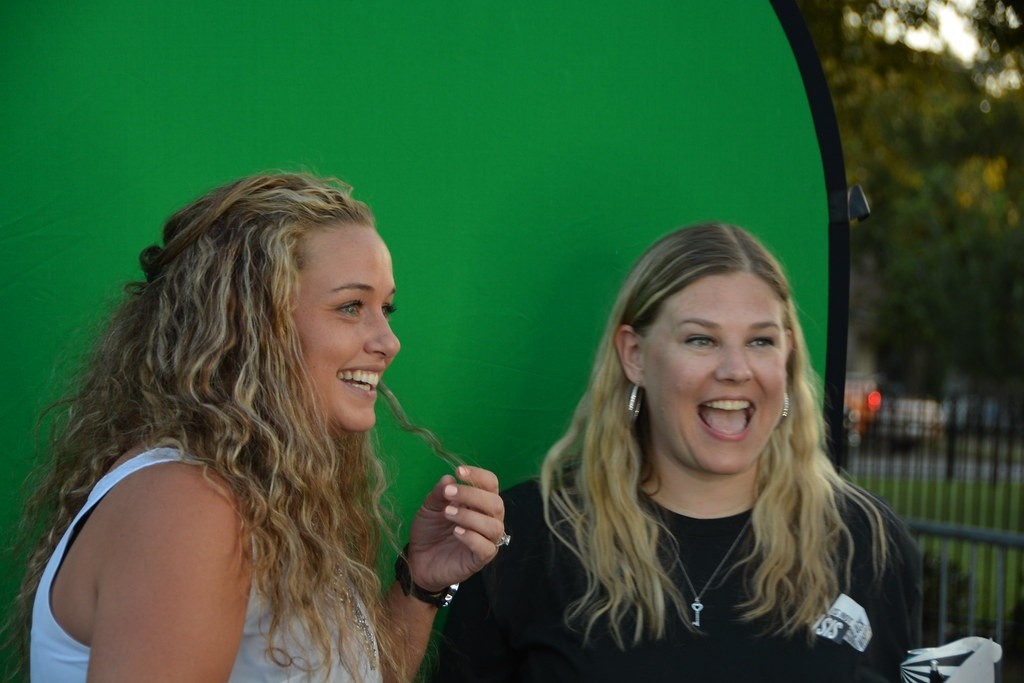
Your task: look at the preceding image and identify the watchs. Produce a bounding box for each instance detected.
[395,542,460,610]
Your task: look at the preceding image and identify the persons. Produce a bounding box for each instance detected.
[417,220,922,683]
[1,175,505,682]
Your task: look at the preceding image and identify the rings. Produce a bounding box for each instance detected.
[496,532,511,549]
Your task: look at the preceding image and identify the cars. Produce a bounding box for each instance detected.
[858,380,943,447]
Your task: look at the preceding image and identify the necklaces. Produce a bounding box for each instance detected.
[650,495,755,627]
[318,560,378,672]
